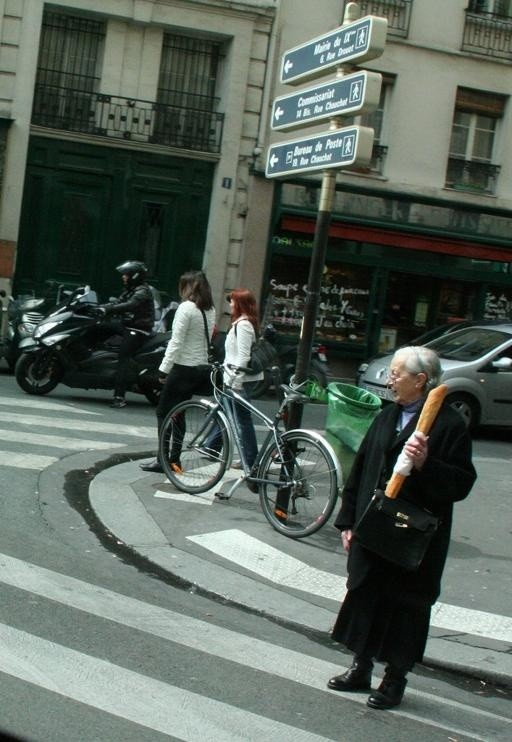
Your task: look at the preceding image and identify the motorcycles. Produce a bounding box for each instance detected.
[13,282,196,407]
[0,285,48,374]
[241,320,331,405]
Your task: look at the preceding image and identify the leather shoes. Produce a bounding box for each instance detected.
[168,461,183,471]
[194,444,220,458]
[137,458,168,474]
[229,461,249,469]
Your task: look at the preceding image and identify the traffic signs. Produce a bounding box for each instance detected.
[265,15,387,181]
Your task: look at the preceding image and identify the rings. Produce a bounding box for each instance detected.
[416,451,421,456]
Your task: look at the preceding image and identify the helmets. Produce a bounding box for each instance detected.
[114,259,148,287]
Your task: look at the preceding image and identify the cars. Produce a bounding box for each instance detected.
[356,318,512,435]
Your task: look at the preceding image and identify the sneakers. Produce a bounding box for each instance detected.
[110,397,129,408]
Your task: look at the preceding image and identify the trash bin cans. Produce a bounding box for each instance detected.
[325,382,382,484]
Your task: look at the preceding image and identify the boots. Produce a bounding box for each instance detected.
[364,669,408,711]
[328,657,373,694]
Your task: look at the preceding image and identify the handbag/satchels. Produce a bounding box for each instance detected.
[207,343,220,364]
[247,336,283,374]
[348,487,443,573]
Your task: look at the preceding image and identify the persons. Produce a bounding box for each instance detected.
[139,270,216,473]
[197,288,264,470]
[93,261,154,408]
[328,346,477,710]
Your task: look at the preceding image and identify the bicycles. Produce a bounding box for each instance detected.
[158,361,344,539]
